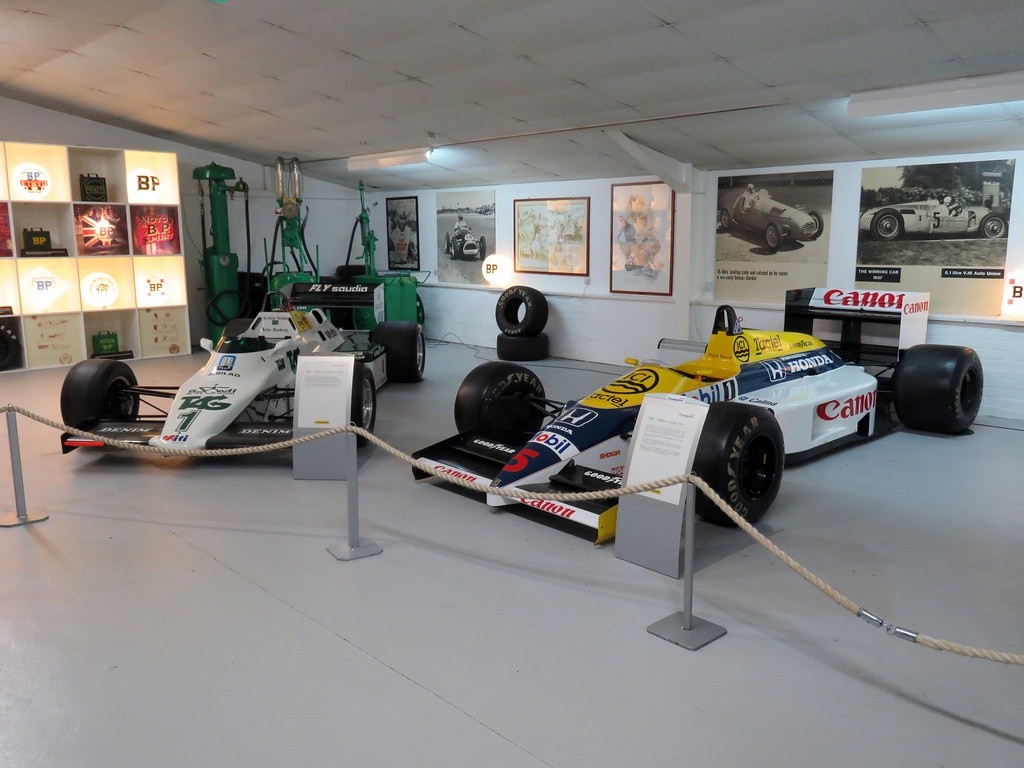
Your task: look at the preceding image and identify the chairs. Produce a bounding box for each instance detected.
[674,305,744,380]
[237,290,298,339]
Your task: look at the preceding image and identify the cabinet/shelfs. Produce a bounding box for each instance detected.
[785,288,929,367]
[0,141,192,373]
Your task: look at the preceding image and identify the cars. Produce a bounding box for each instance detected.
[859,195,1008,241]
[718,187,824,252]
[444,224,487,260]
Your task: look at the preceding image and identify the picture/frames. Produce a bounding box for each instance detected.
[611,181,675,296]
[386,196,420,271]
[514,198,590,276]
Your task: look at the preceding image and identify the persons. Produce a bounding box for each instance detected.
[454,214,472,231]
[388,207,418,268]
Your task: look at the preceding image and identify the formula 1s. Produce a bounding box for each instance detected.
[412,285,984,546]
[60,273,429,456]
